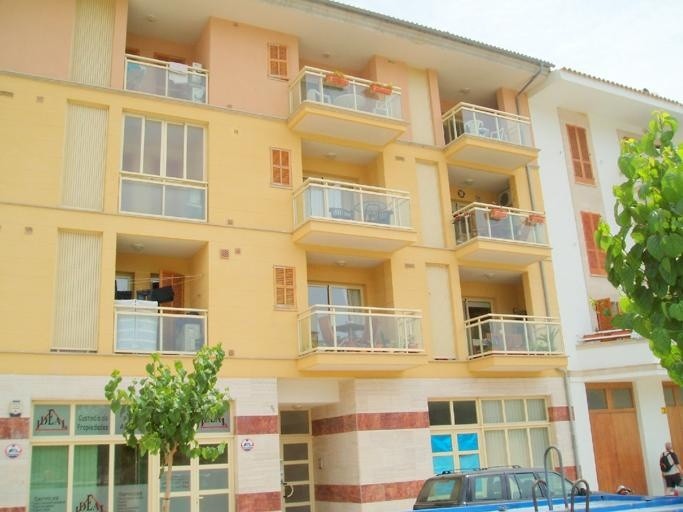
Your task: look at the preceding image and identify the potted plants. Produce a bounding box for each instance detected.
[535,329,560,355]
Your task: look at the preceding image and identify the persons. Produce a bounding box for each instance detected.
[615,484,627,495]
[659,441,683,496]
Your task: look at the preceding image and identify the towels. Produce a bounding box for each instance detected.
[169,62,188,85]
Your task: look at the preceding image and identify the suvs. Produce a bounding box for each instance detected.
[414,463,612,511]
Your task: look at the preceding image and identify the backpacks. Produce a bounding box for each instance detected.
[659,452,675,472]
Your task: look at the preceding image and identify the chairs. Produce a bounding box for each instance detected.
[318,312,401,353]
[460,114,515,142]
[327,194,398,225]
[492,479,542,499]
[306,89,402,118]
